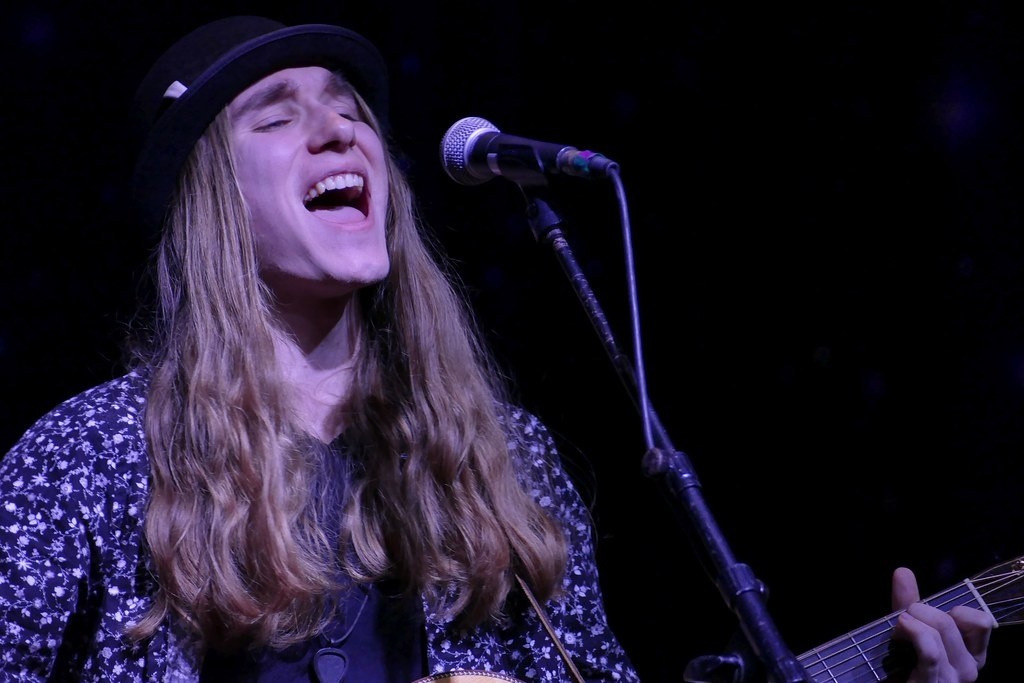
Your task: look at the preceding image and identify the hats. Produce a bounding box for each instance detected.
[121,16,387,221]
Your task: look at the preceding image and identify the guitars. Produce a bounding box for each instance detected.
[407,554,1024,683]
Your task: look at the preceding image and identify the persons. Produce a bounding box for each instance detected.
[0,15,994,682]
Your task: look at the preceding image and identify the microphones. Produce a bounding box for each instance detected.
[439,116,621,187]
